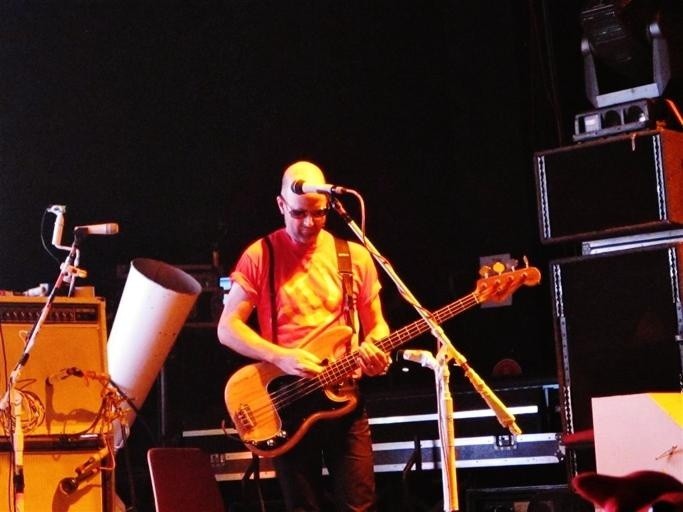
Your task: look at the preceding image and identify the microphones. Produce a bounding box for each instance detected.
[48,366,83,384]
[291,179,344,195]
[63,467,98,490]
[74,222,119,235]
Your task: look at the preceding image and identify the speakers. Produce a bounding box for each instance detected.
[0,296,115,447]
[0,447,115,512]
[532,128,683,245]
[548,241,683,484]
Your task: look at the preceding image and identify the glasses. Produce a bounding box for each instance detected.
[280,194,331,221]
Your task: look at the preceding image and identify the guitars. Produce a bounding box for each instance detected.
[224,257,543,461]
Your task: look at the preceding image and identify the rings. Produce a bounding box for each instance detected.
[366,362,374,369]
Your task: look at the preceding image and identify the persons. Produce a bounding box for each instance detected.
[213,160,393,511]
